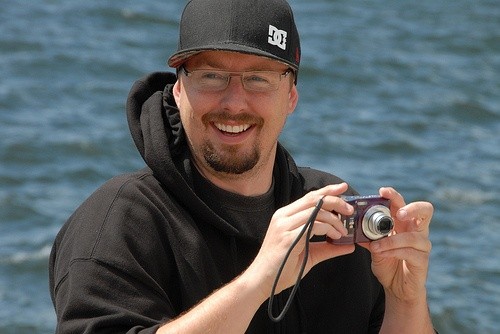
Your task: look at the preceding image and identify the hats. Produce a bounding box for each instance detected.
[167,0,301,71]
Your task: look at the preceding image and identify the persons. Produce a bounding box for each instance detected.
[48,0,439,334]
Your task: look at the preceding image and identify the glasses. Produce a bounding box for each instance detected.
[181,63,291,92]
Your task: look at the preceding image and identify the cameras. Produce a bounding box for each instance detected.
[326,194,395,245]
